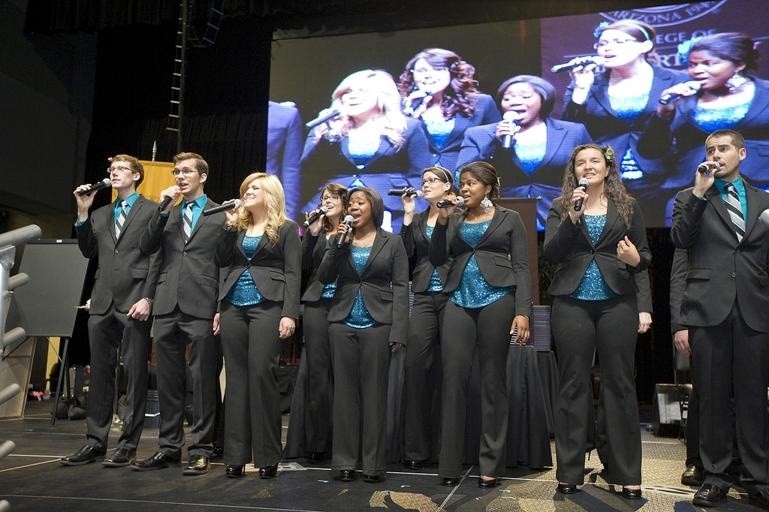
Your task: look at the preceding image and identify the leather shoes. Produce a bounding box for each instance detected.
[692,479,729,505]
[746,487,768,510]
[128,449,181,473]
[58,443,108,466]
[180,453,212,477]
[678,462,705,486]
[101,445,138,468]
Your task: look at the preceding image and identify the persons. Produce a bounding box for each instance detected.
[388,166,463,471]
[398,48,500,181]
[637,32,769,225]
[672,244,706,485]
[454,75,594,232]
[558,18,697,192]
[544,143,652,499]
[300,185,350,463]
[130,152,227,474]
[299,69,434,237]
[263,100,302,225]
[430,162,533,487]
[59,155,164,467]
[314,186,410,484]
[670,130,769,506]
[219,172,302,478]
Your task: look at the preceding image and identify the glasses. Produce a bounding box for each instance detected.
[320,195,342,202]
[170,168,204,177]
[418,176,442,187]
[105,166,140,175]
[593,38,641,49]
[409,66,448,75]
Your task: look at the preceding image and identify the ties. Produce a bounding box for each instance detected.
[181,199,197,242]
[113,200,129,241]
[723,181,748,251]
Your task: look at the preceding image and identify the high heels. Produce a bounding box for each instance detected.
[622,481,643,500]
[224,463,247,480]
[556,481,577,495]
[442,477,455,487]
[259,465,277,479]
[479,474,497,490]
[405,459,421,470]
[363,474,378,485]
[340,468,355,483]
[307,450,324,465]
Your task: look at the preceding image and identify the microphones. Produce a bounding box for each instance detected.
[203,198,241,216]
[502,111,518,149]
[658,81,703,105]
[158,187,181,212]
[698,160,720,174]
[550,56,604,74]
[388,189,423,199]
[302,207,329,228]
[405,86,431,117]
[79,178,112,196]
[574,177,589,212]
[436,196,464,208]
[336,215,354,248]
[305,107,343,128]
